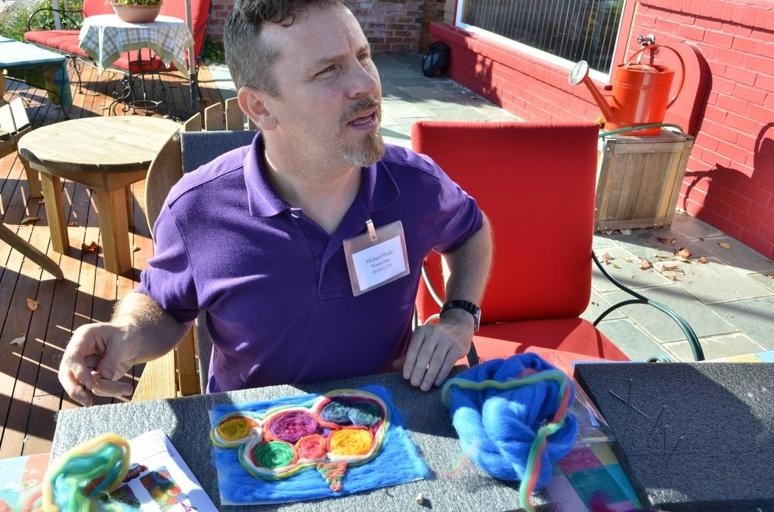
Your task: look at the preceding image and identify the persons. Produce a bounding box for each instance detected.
[58,1,493,407]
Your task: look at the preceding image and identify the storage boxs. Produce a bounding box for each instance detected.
[594,126,696,233]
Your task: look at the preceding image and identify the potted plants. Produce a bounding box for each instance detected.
[107,1,165,23]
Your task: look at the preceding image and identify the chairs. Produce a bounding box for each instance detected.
[21,0,214,115]
[411,120,706,379]
[1,74,68,201]
[177,128,259,395]
[142,96,260,394]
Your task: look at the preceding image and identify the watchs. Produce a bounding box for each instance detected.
[439,299,481,332]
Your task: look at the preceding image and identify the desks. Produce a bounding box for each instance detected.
[76,12,196,111]
[16,114,185,276]
[0,36,68,124]
[573,360,774,511]
[45,361,550,512]
